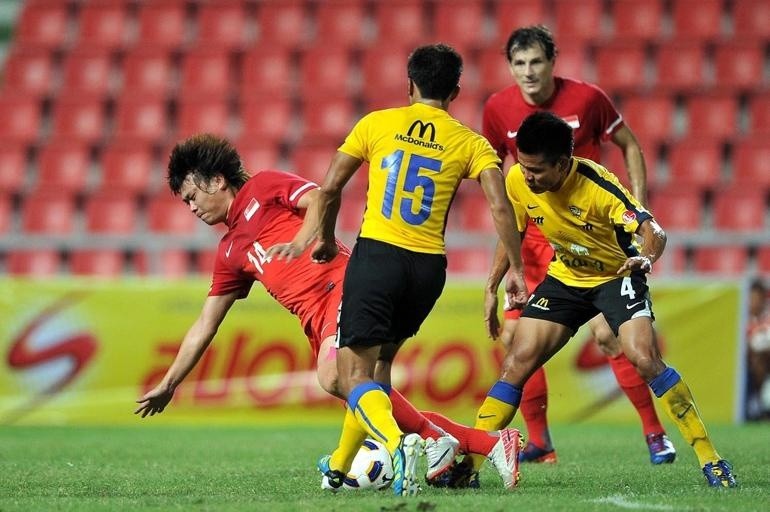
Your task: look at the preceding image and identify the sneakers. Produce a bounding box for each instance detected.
[421,425,486,491]
[515,435,559,467]
[644,428,678,466]
[701,456,738,489]
[317,451,348,491]
[485,427,524,489]
[390,429,429,499]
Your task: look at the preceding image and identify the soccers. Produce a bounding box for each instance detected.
[343,439,394,491]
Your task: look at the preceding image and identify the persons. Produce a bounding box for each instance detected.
[741,271,770,422]
[484,23,676,465]
[435,109,737,488]
[134,133,520,488]
[309,42,527,496]
